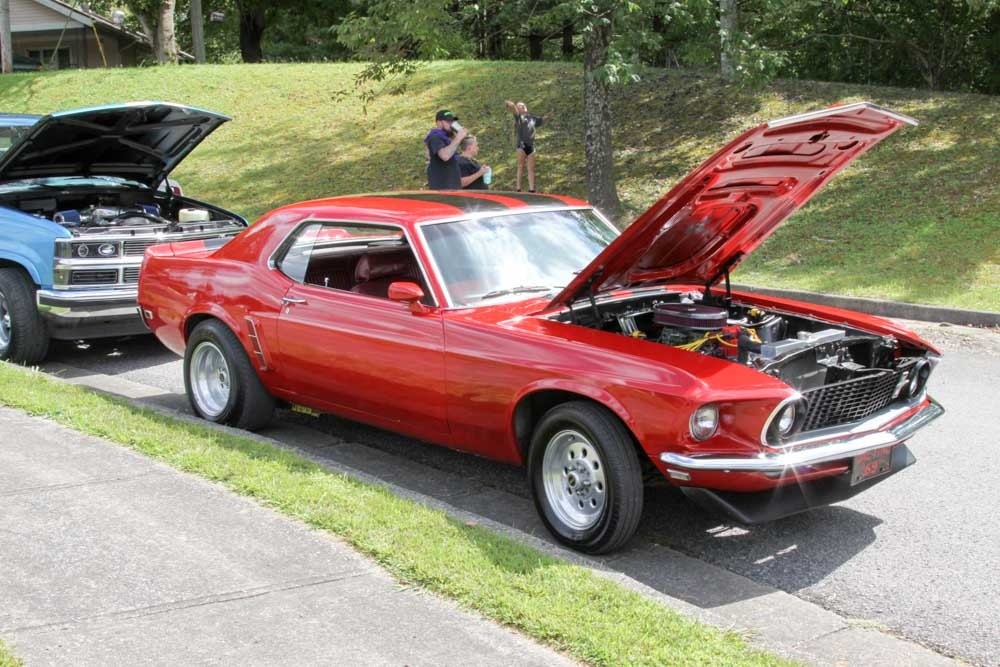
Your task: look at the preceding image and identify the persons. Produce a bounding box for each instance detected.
[458,135,489,190]
[424,109,468,190]
[505,100,554,193]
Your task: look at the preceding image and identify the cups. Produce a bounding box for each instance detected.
[451,120,462,131]
[483,167,492,185]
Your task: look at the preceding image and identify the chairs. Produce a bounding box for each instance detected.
[309,245,510,298]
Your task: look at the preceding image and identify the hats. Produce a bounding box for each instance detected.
[436,109,458,121]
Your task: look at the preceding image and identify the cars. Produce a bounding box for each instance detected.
[136,100,945,558]
[0,100,251,370]
